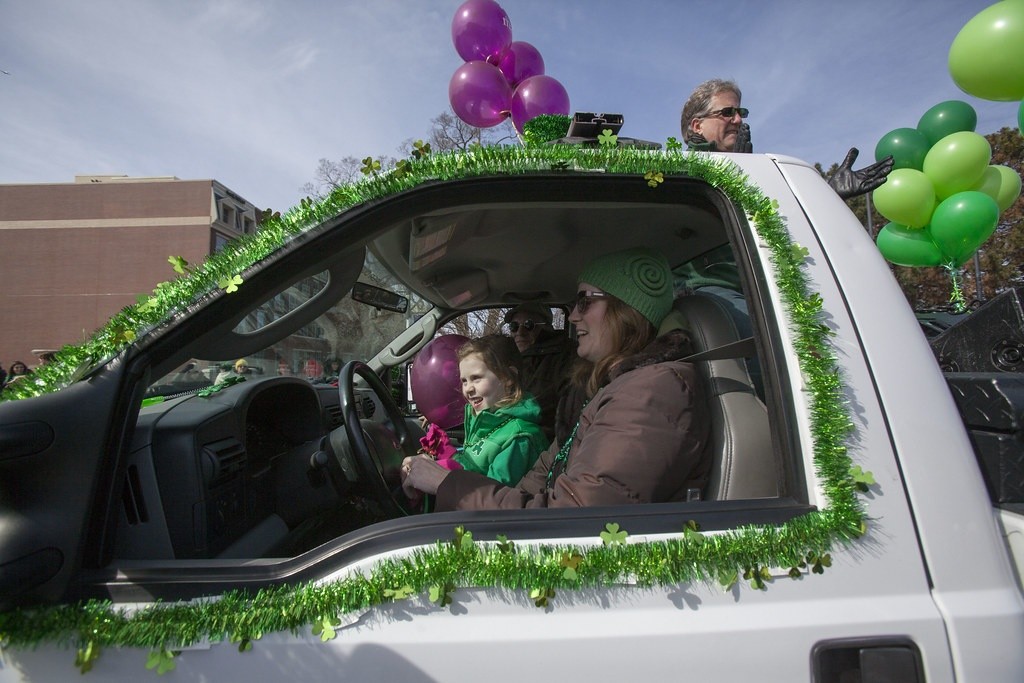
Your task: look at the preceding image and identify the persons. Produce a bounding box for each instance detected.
[429,334,551,489]
[0,350,347,396]
[400,245,710,513]
[504,298,587,446]
[679,77,897,199]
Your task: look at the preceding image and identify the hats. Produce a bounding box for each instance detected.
[577,246,674,331]
[236,359,247,371]
[504,302,553,323]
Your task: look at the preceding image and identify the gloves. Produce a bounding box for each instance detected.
[734,123,753,153]
[827,147,894,201]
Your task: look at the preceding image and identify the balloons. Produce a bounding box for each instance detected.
[411,334,480,432]
[450,0,571,138]
[947,0,1024,102]
[872,100,1024,270]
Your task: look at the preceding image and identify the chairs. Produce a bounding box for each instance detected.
[673,292,777,498]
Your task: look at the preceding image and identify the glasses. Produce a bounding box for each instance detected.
[578,291,612,313]
[507,319,547,332]
[688,107,750,125]
[239,366,246,368]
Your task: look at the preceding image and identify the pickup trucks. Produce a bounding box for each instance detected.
[2,141,1024,683]
